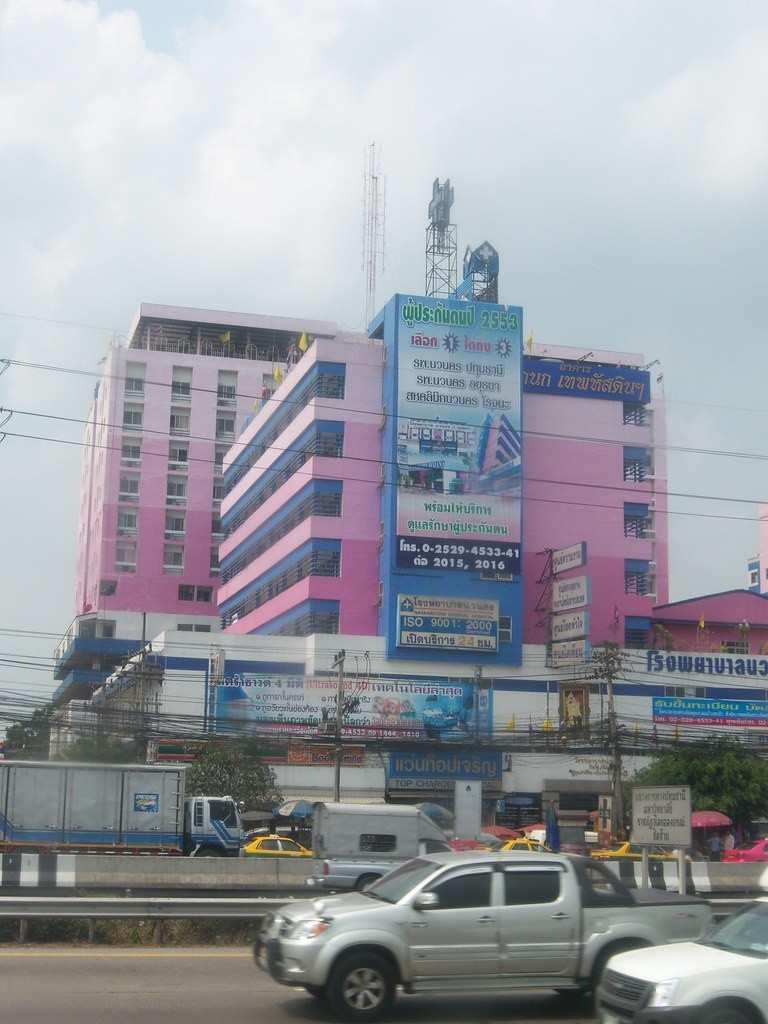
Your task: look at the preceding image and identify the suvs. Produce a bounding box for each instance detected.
[593,867,768,1024]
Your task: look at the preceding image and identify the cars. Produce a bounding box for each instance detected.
[239,833,313,859]
[444,830,552,853]
[590,839,692,862]
[722,838,768,864]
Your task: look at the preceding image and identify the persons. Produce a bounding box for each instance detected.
[287,826,299,839]
[706,827,738,861]
[373,697,415,719]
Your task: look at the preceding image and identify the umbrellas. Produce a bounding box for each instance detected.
[482,823,546,839]
[414,803,454,819]
[272,800,314,826]
[692,811,732,827]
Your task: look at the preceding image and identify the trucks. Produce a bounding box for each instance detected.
[311,801,457,862]
[0,758,246,857]
[554,809,589,854]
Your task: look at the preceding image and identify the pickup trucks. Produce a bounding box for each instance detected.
[252,850,713,1024]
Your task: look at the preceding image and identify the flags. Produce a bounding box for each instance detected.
[220,332,230,342]
[526,336,532,351]
[254,399,258,414]
[274,367,282,384]
[299,333,308,351]
